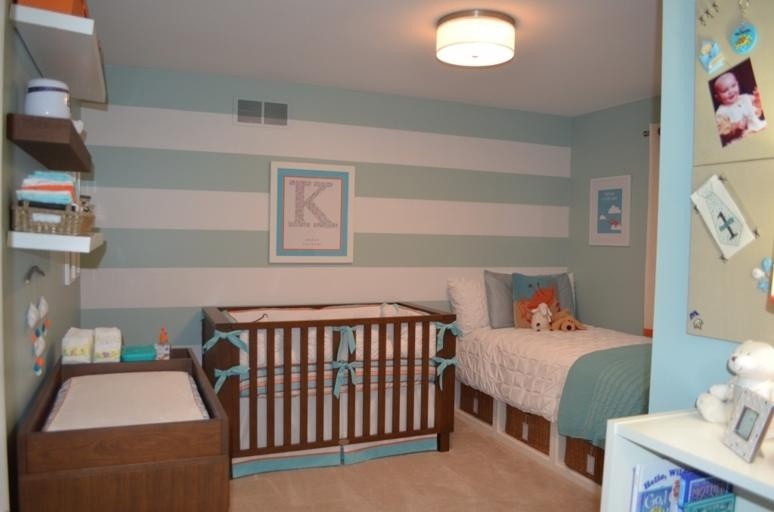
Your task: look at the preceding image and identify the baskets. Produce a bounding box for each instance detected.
[11,199,95,238]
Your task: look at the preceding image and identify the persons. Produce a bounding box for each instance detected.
[712,72,765,144]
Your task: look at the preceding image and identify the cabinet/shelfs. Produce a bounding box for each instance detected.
[4,0,107,253]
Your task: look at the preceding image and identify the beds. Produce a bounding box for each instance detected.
[456,318,651,496]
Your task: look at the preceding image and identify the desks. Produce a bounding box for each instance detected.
[598,409,774,512]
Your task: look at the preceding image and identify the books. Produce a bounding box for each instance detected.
[626,457,732,512]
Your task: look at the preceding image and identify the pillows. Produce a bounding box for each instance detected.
[443,269,576,331]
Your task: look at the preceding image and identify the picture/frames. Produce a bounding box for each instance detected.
[588,174,632,248]
[268,160,356,263]
[720,389,774,466]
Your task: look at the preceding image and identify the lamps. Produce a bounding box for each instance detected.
[434,9,515,67]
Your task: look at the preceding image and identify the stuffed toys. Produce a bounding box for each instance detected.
[549,308,585,332]
[529,303,551,330]
[696,339,774,424]
